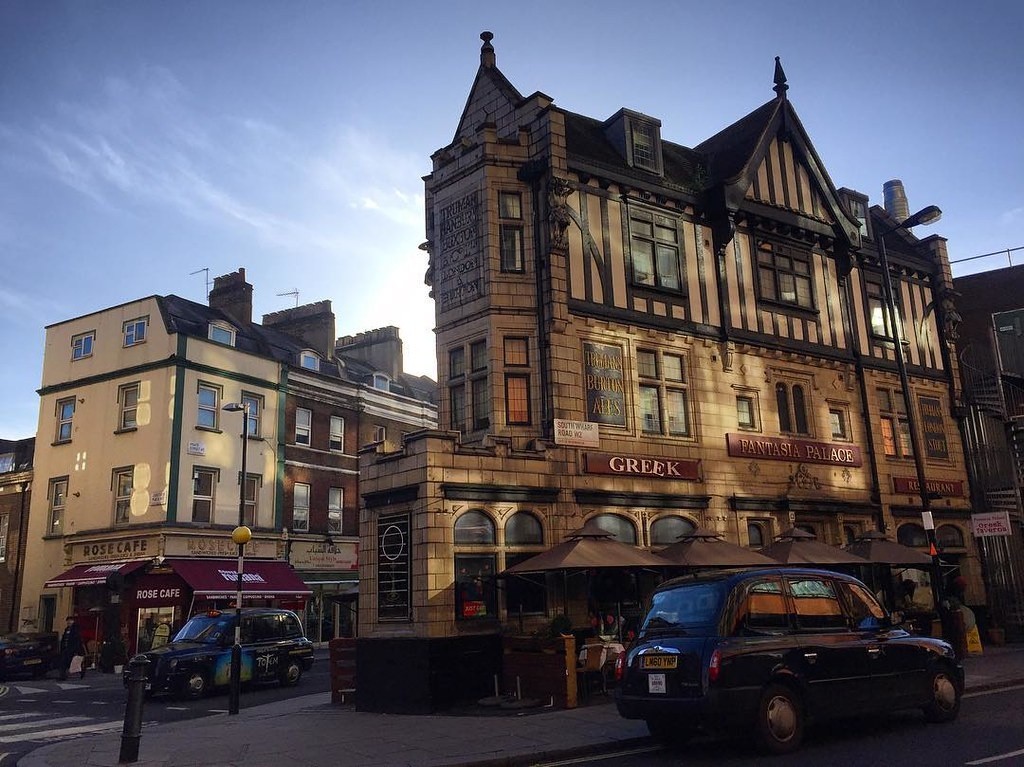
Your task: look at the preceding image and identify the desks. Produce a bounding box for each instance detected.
[578,640,624,669]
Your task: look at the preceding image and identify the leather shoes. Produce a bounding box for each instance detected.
[56,677,65,682]
[80,669,86,680]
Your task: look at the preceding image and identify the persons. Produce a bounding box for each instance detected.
[57,615,88,681]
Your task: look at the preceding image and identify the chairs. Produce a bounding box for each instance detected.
[575,644,608,702]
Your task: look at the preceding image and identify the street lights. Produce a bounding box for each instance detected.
[221,400,251,714]
[875,206,964,675]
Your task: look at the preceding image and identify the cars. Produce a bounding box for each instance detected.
[614,563,964,754]
[1,632,53,680]
[125,606,313,701]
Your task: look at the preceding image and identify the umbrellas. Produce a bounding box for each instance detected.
[751,527,872,571]
[643,526,782,585]
[830,530,944,615]
[489,523,676,645]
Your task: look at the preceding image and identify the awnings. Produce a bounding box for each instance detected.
[42,560,151,591]
[167,558,315,602]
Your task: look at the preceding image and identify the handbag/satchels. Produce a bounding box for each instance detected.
[69,656,84,673]
